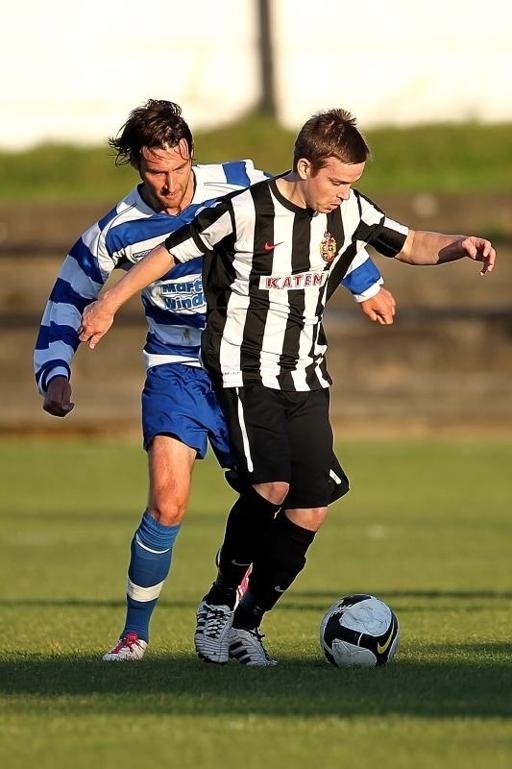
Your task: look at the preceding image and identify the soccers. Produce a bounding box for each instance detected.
[317,588,401,669]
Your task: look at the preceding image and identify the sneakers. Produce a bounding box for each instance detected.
[192,593,237,668]
[100,632,150,662]
[228,622,279,669]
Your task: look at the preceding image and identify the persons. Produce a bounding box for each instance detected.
[77,108,497,668]
[34,99,397,660]
